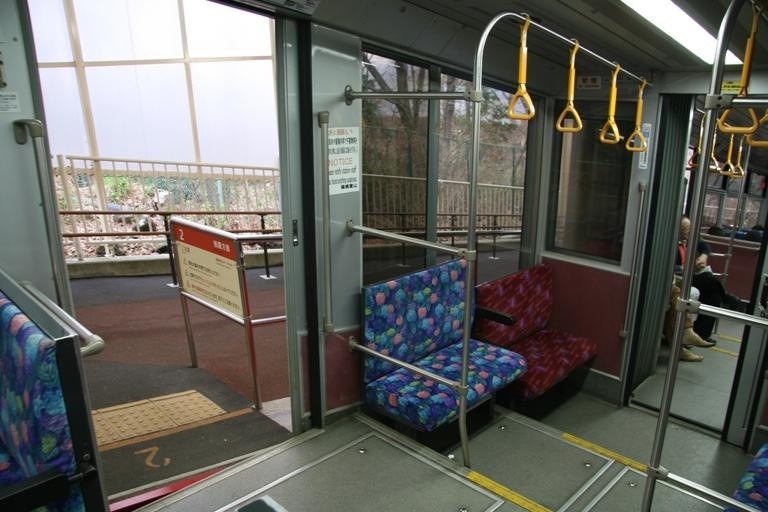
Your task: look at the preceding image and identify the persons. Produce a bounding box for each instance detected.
[667,215,764,362]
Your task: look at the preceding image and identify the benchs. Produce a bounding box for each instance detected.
[359,255,527,442]
[470,264,599,412]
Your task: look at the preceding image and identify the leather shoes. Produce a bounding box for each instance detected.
[666,327,716,362]
